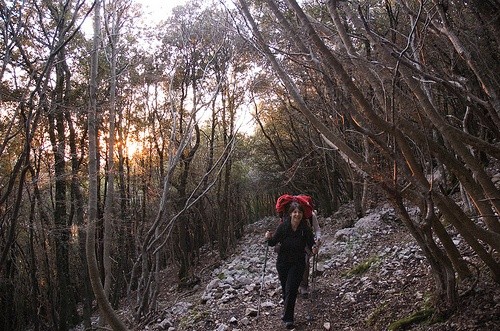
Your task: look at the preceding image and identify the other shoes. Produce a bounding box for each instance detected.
[280,310,288,320]
[285,322,296,331]
[299,286,308,297]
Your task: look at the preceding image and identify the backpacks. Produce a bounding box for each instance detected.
[275,193,313,225]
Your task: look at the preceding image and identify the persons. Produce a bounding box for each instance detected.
[265,202,314,328]
[296,194,323,297]
